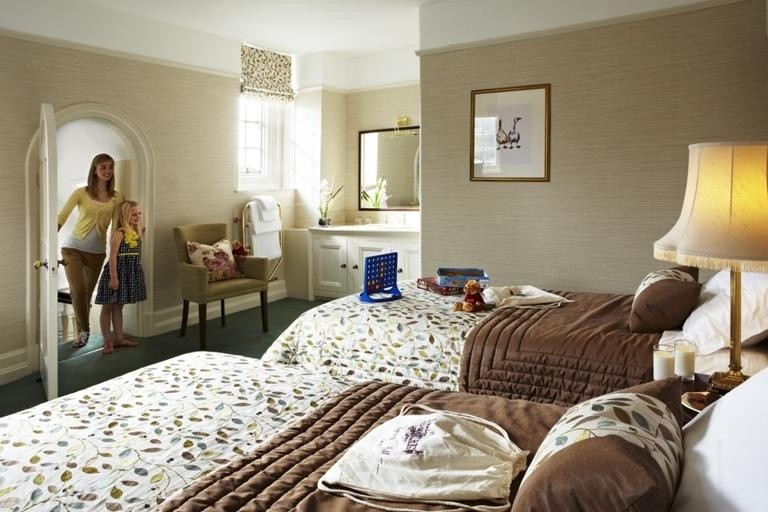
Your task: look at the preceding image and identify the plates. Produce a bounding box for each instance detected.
[679,389,725,413]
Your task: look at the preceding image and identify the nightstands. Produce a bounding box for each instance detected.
[670,369,757,425]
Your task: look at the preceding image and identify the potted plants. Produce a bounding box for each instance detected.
[358,174,395,209]
[314,175,345,227]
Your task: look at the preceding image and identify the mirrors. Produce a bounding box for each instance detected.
[357,125,421,212]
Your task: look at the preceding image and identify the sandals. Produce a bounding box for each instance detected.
[73,332,89,348]
[114,338,136,347]
[103,336,114,354]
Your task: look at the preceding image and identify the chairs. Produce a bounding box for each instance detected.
[172,222,271,352]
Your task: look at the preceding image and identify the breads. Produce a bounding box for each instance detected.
[687,391,721,410]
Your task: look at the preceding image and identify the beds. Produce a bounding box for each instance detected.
[0,343,768,511]
[260,262,767,411]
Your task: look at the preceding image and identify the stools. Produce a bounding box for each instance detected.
[56,284,82,346]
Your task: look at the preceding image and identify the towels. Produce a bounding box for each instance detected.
[248,226,283,261]
[256,200,280,221]
[249,203,283,234]
[251,192,278,211]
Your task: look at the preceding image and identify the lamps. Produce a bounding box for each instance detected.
[650,140,768,397]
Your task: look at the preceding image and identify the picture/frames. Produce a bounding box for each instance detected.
[468,83,551,183]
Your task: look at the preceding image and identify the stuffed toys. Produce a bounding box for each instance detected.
[453,281,486,312]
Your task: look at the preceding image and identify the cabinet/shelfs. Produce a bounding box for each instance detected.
[311,236,391,299]
[390,240,421,284]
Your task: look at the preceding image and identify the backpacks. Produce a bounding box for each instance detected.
[318,403,529,512]
[483,285,576,309]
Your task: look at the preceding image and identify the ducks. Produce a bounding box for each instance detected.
[508,117,522,149]
[495,119,508,150]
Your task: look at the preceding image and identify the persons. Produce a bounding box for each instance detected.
[53,153,129,348]
[99,200,146,353]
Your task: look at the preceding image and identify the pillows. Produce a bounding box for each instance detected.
[183,236,245,283]
[510,372,684,511]
[682,263,768,359]
[670,364,768,511]
[627,263,708,335]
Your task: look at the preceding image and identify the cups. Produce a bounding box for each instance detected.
[671,338,697,382]
[354,215,361,226]
[364,216,371,224]
[652,343,676,382]
